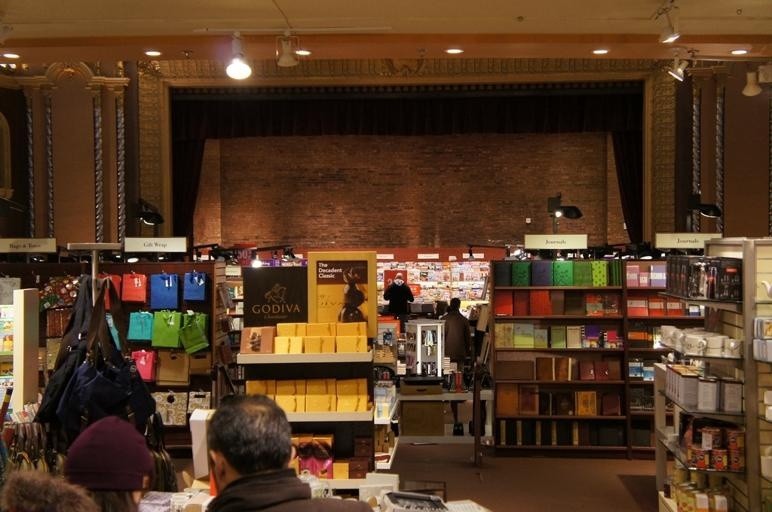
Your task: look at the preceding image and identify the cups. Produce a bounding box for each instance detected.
[661,326,742,358]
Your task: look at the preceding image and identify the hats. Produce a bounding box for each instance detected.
[63,414,155,491]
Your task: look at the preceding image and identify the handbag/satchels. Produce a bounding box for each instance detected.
[155,349,190,387]
[151,307,186,350]
[184,270,207,303]
[188,391,214,414]
[127,311,151,344]
[34,273,97,425]
[182,309,209,332]
[56,276,155,434]
[190,350,213,377]
[149,272,180,310]
[131,351,155,385]
[149,391,188,428]
[118,272,147,303]
[97,271,121,307]
[177,322,209,355]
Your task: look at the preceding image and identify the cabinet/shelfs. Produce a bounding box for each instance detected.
[0,288,40,414]
[478,258,631,460]
[235,345,374,506]
[626,259,706,451]
[653,235,772,512]
[403,318,446,386]
[41,260,233,449]
[374,349,399,471]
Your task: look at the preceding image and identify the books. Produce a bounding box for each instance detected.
[628,321,652,348]
[632,418,652,447]
[551,326,566,348]
[494,262,511,286]
[595,359,610,381]
[512,262,530,286]
[666,296,683,316]
[604,295,623,316]
[592,260,608,287]
[619,262,622,286]
[653,326,663,349]
[550,291,564,314]
[650,264,667,288]
[616,261,619,286]
[493,292,513,316]
[627,265,639,288]
[573,261,592,287]
[609,260,615,286]
[530,290,550,315]
[497,384,621,416]
[627,294,649,316]
[494,360,533,379]
[585,293,603,316]
[514,291,528,316]
[579,359,595,381]
[535,329,547,348]
[495,420,627,447]
[495,324,513,348]
[554,261,573,286]
[531,260,553,286]
[640,272,650,287]
[649,294,665,316]
[536,357,553,381]
[555,356,572,381]
[689,305,700,316]
[643,367,654,381]
[567,326,581,347]
[513,323,534,348]
[629,361,644,378]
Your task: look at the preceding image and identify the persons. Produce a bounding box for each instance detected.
[441,298,471,362]
[384,272,414,314]
[1,415,155,512]
[207,394,374,512]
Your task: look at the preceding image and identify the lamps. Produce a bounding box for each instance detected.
[649,0,682,45]
[273,31,301,69]
[667,45,690,82]
[225,31,253,82]
[740,63,763,97]
